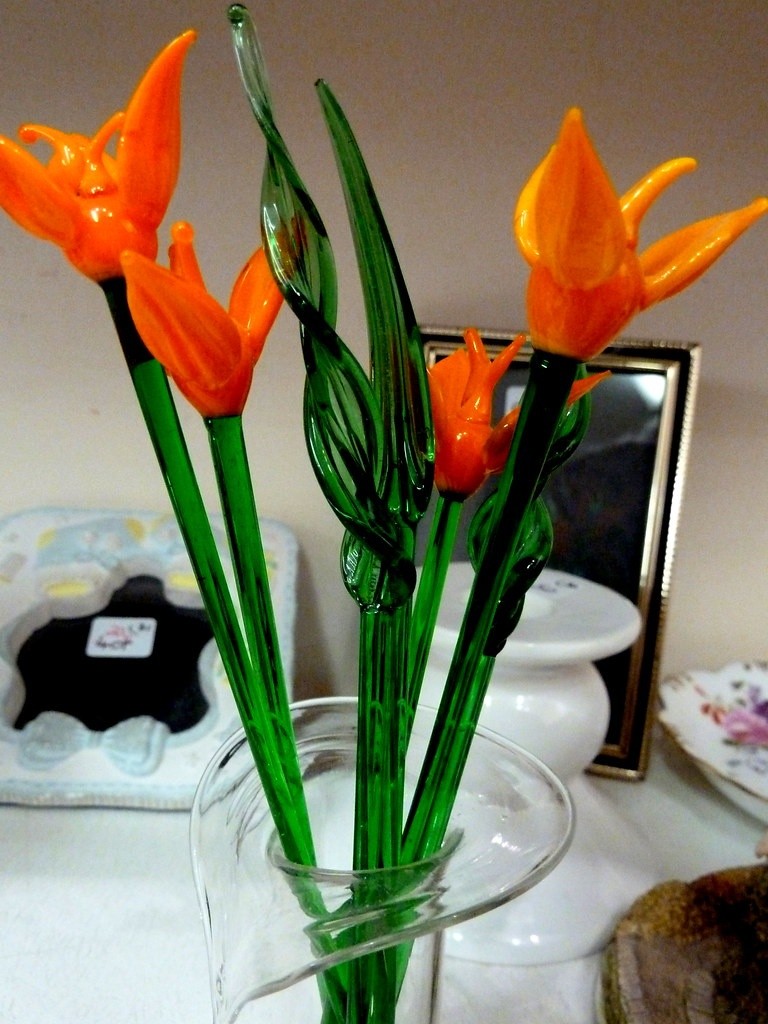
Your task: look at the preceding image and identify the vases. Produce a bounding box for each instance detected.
[193,691,579,1024]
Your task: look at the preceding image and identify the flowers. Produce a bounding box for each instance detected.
[0,0,768,1024]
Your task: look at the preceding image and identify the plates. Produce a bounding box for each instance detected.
[659,659,768,824]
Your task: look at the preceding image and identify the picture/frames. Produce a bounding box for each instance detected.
[417,325,699,781]
